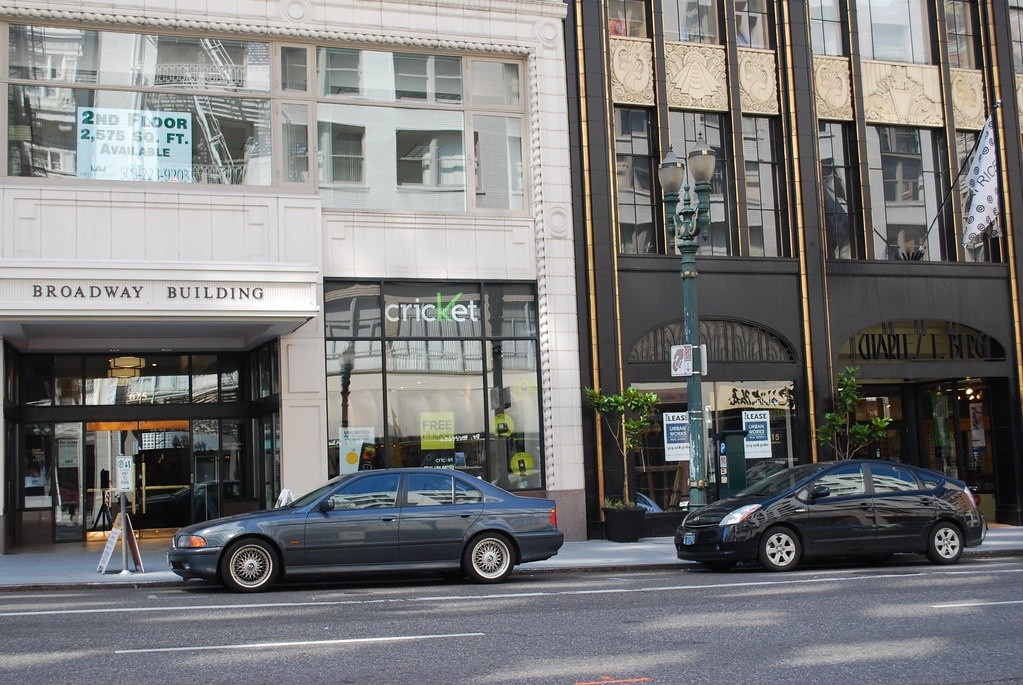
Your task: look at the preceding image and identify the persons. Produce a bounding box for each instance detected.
[66,486,77,521]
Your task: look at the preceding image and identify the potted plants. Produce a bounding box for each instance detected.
[582,385,662,543]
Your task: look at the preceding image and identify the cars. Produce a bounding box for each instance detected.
[746,457,798,481]
[637,492,663,515]
[674,459,988,572]
[129,481,246,529]
[167,468,564,593]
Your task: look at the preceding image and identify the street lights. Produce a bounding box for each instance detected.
[657,130,715,515]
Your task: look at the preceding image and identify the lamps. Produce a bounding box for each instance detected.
[966,394,975,400]
[108,368,140,378]
[965,387,974,394]
[976,390,982,400]
[108,357,145,368]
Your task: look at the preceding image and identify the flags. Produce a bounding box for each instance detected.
[962,113,1003,249]
